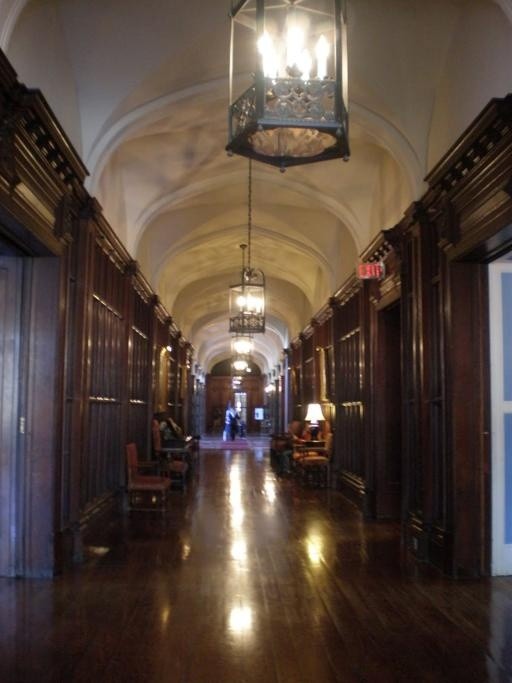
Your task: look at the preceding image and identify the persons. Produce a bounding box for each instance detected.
[222,399,240,442]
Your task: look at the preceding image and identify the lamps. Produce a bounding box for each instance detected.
[217,1,352,175]
[302,402,328,443]
[227,142,267,333]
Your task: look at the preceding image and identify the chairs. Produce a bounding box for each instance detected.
[268,419,338,489]
[122,415,202,520]
